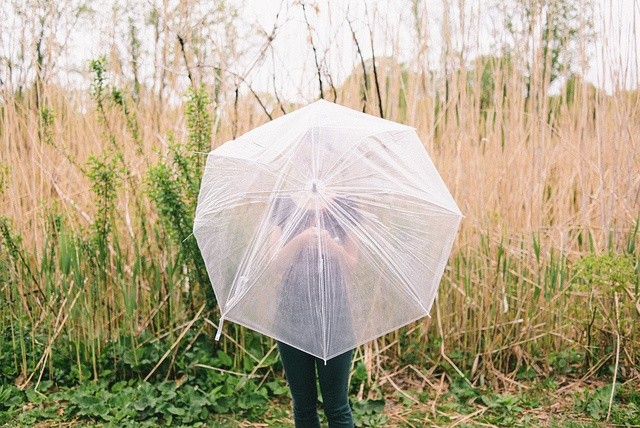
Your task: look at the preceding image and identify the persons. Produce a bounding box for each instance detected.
[264,187,365,427]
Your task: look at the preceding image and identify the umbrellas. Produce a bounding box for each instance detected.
[194,96,466,365]
